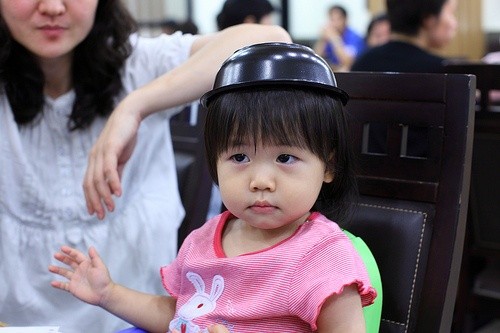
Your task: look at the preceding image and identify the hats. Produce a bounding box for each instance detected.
[199,42,350,109]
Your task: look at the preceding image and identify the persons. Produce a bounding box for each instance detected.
[49,42,377,333]
[349,0,492,73]
[314,5,362,63]
[364,15,390,47]
[0,0,294,333]
[215,0,347,72]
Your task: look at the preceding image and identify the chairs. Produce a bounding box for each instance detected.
[182,71,476,333]
